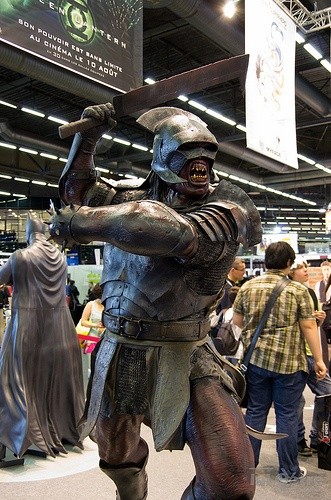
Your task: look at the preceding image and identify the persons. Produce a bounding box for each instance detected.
[0,217,85,460]
[233,241,327,484]
[209,258,331,457]
[0,278,106,379]
[49,102,264,500]
